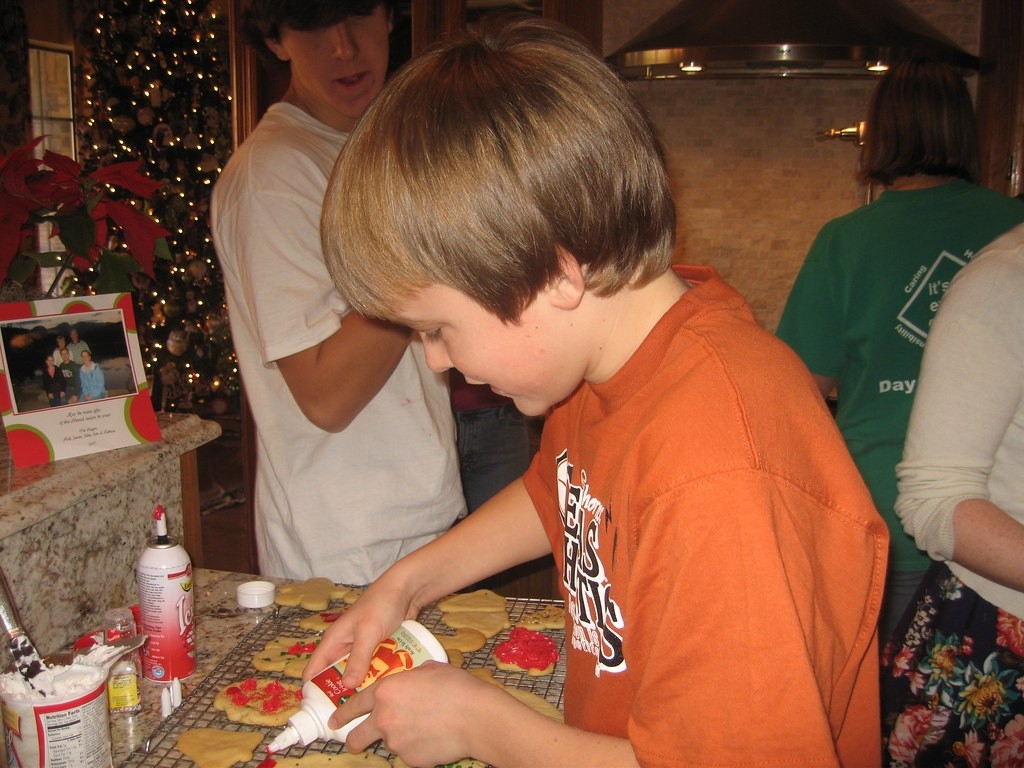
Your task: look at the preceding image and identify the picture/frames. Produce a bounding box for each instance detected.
[0,292,163,470]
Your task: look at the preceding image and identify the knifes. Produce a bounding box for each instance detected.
[0,566,53,698]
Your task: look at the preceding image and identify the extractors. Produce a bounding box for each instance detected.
[604,0,988,80]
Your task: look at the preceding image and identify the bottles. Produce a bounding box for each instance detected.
[103,608,145,714]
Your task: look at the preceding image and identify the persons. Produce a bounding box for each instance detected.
[209,0,472,589]
[41,327,108,407]
[301,21,890,768]
[775,42,1024,648]
[879,224,1024,768]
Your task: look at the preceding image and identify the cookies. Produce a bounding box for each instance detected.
[175,576,567,768]
